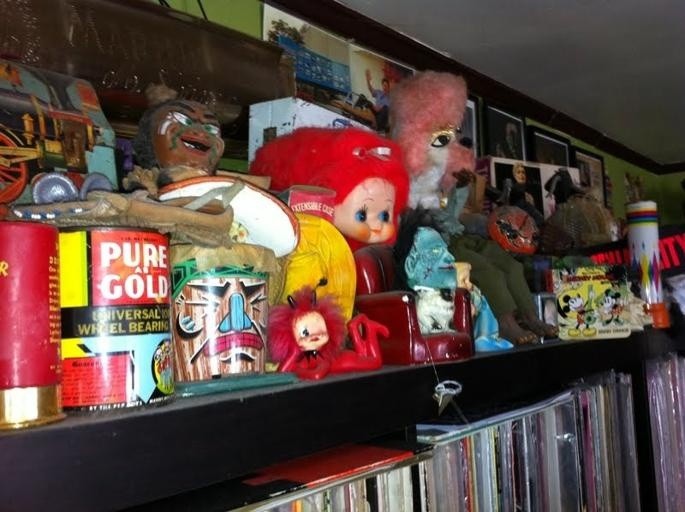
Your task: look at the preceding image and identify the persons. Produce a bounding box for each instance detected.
[502,163,535,211]
[270,289,390,378]
[352,68,391,134]
[251,126,410,290]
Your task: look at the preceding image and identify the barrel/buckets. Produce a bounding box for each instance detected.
[58,225,178,411]
[167,242,276,381]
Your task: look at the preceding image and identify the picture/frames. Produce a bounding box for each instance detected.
[452,93,608,209]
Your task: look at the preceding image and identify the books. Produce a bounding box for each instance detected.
[233,351,684,511]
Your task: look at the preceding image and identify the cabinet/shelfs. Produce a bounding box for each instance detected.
[1,311,685,512]
